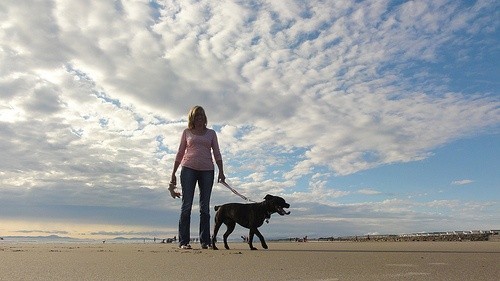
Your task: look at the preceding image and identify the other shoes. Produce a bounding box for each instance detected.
[178,244,192,250]
[201,243,212,249]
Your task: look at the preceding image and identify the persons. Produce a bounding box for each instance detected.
[168,105,224,249]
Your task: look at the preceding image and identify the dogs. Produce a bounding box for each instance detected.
[211,194,291,251]
